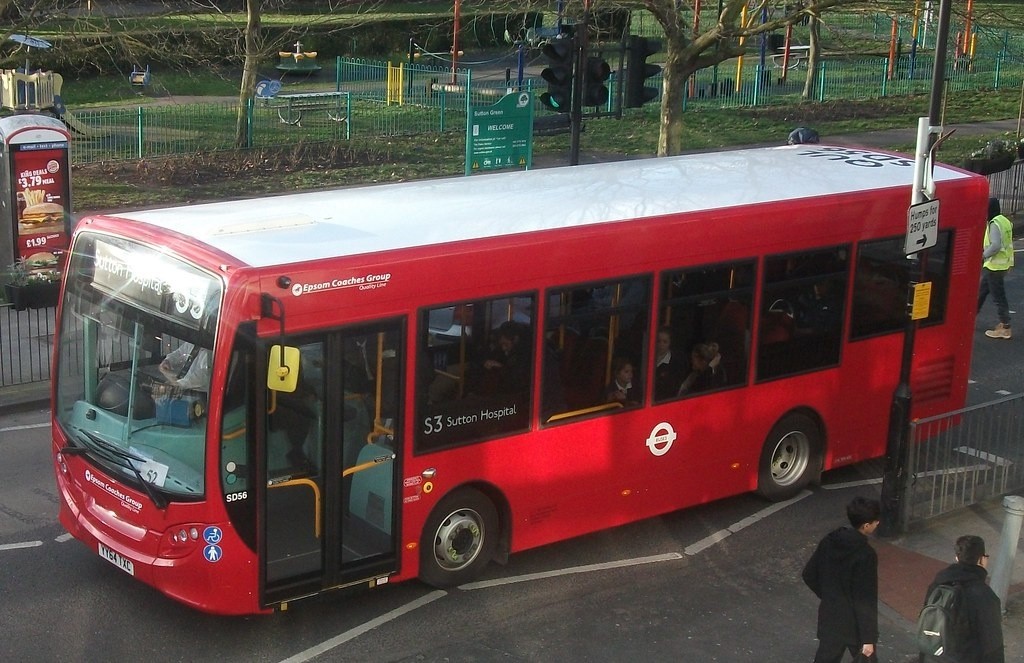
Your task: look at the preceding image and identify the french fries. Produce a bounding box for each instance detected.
[23,187,45,206]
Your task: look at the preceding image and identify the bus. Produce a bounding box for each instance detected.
[48,145,988,617]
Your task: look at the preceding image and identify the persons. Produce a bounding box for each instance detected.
[802,496,880,663]
[656,326,679,388]
[764,256,841,372]
[600,361,637,407]
[977,197,1015,339]
[159,342,213,402]
[468,321,533,399]
[686,344,736,394]
[919,535,1005,663]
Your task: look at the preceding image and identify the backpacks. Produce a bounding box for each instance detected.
[916,582,988,663]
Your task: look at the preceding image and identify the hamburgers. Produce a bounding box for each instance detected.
[19,203,64,228]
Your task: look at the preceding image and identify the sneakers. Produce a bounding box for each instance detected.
[985,323,1012,339]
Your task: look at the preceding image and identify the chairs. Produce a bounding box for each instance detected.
[270,251,944,492]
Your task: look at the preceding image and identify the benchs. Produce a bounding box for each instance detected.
[290,106,347,127]
[266,102,336,123]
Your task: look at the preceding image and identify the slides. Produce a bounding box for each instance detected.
[52,72,112,138]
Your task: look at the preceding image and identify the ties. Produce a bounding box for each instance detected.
[176,345,201,379]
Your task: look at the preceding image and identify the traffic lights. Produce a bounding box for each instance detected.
[584,57,610,107]
[541,38,575,112]
[624,35,661,107]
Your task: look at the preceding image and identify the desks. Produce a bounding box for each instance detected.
[256,90,347,125]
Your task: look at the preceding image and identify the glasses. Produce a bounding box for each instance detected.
[980,552,990,559]
